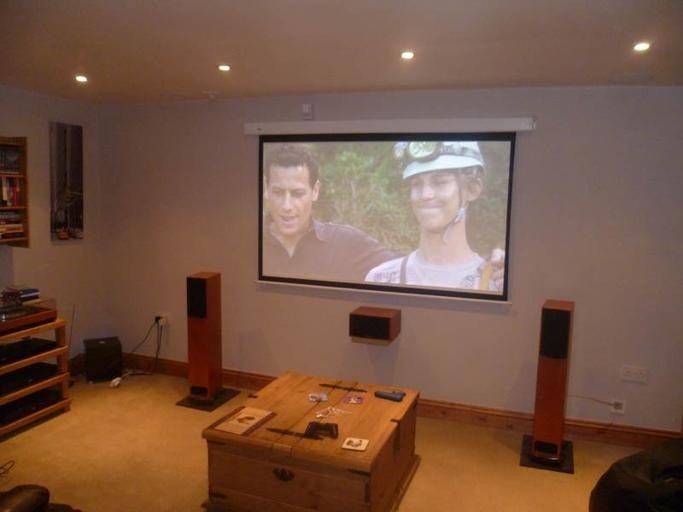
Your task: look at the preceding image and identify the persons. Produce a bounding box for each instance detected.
[263,142,505,296]
[362,141,504,295]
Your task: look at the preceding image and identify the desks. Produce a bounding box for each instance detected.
[201,370,423,512]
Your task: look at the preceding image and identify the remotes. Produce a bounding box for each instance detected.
[376,390,401,401]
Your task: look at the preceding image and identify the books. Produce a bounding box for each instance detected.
[0,144,27,239]
[6,284,42,306]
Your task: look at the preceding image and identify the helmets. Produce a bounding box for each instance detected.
[392,140,485,181]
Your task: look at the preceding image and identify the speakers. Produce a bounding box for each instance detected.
[349,307,400,345]
[185,271,225,406]
[527,299,576,466]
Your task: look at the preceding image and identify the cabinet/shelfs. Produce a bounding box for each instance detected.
[0,136,30,248]
[0,317,72,436]
